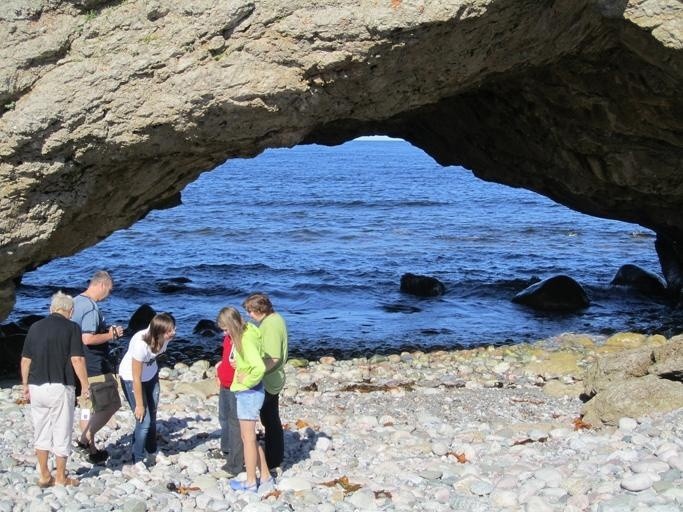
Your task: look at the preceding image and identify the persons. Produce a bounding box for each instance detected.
[67,270,124,466]
[217,306,275,495]
[246,293,289,469]
[19,289,92,488]
[118,314,176,462]
[208,334,245,478]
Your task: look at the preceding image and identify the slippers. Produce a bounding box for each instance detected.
[55,478,79,487]
[37,475,55,488]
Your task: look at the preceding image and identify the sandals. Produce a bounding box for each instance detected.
[256,476,274,485]
[230,480,258,492]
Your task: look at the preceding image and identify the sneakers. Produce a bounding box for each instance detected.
[207,450,228,458]
[89,449,108,462]
[216,470,235,478]
[131,460,150,482]
[78,441,90,449]
[147,453,172,466]
